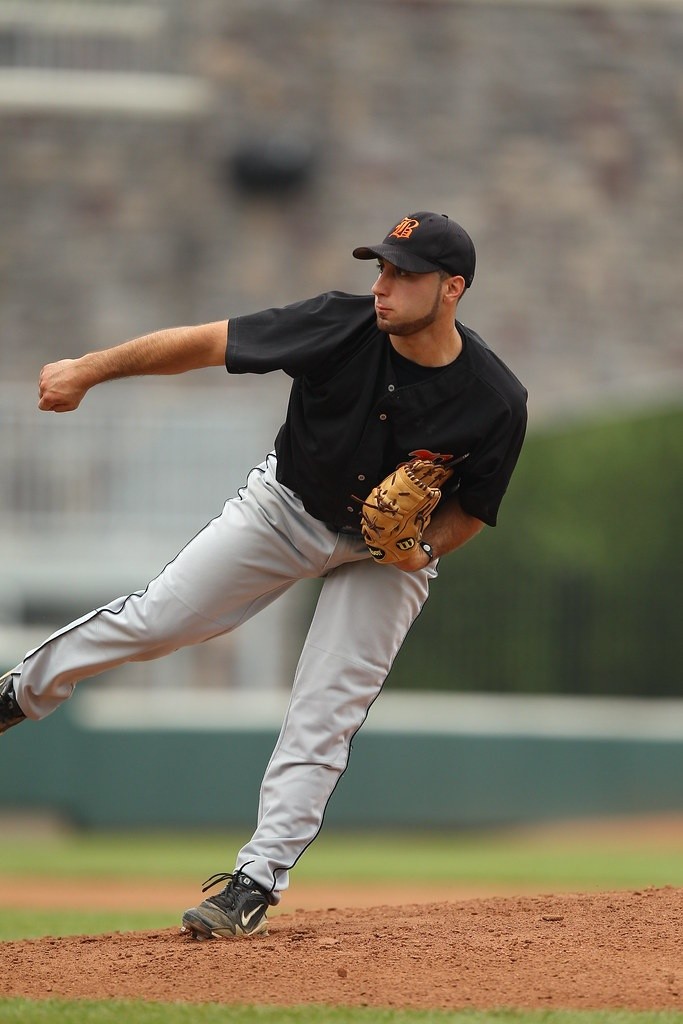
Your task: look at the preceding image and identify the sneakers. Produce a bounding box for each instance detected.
[182,860,271,940]
[0,671,27,735]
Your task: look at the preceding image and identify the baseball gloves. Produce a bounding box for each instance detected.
[362,460,453,565]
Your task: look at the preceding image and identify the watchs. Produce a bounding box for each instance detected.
[420,542,434,566]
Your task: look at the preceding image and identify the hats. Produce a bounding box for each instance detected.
[353,211,476,288]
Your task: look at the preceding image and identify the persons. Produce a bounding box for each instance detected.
[0,213,528,941]
